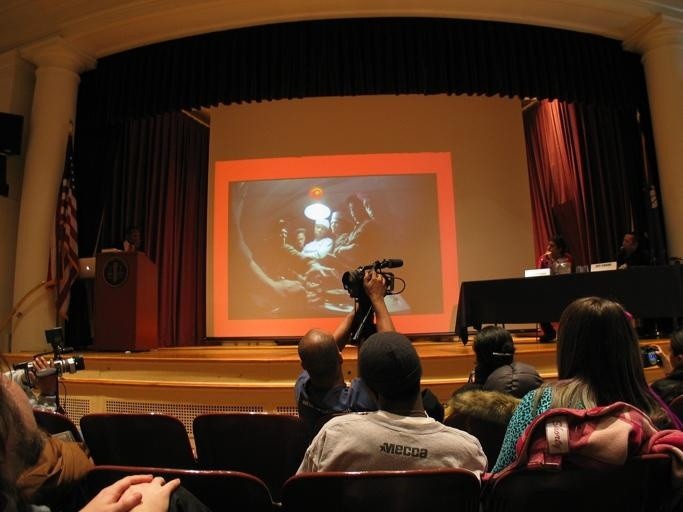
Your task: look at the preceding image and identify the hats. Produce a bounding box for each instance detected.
[357,329,425,396]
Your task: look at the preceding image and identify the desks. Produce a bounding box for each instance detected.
[454,264,683,340]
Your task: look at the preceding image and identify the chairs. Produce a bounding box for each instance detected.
[29,408,674,511]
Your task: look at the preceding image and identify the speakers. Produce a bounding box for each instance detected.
[0,112,24,156]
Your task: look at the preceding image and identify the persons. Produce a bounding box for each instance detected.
[536,236,573,343]
[440,324,683,471]
[232,183,385,312]
[0,354,205,511]
[296,331,489,473]
[608,232,658,340]
[115,227,139,251]
[293,272,396,419]
[493,295,683,470]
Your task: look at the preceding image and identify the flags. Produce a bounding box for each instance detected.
[45,130,80,323]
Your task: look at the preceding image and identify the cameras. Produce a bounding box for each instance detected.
[640,344,661,368]
